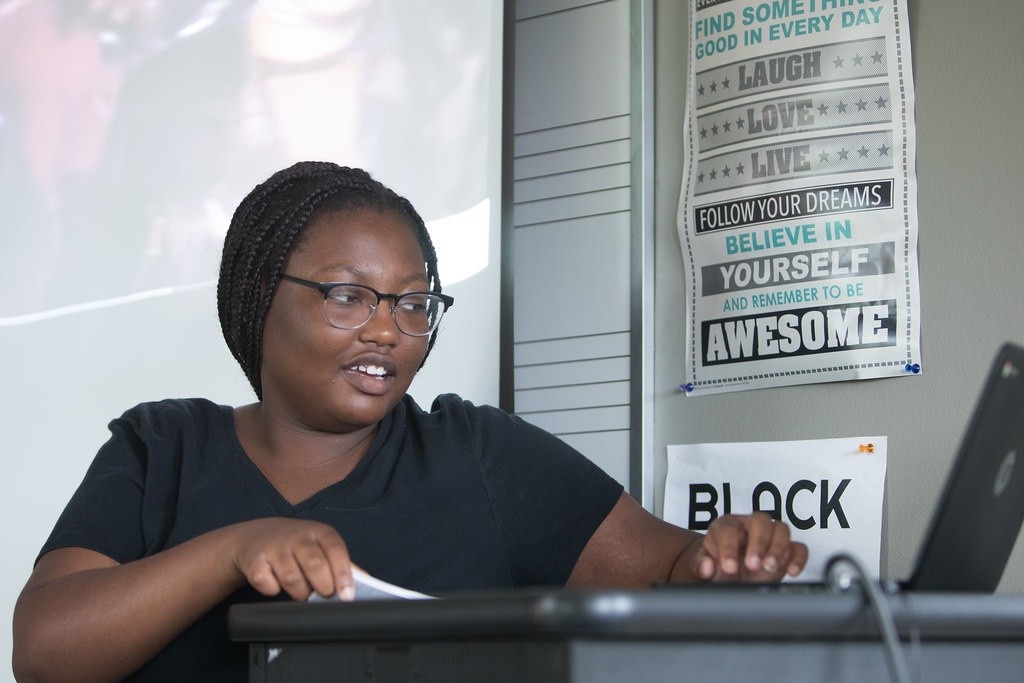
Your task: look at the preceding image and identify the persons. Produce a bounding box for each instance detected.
[11,160,808,683]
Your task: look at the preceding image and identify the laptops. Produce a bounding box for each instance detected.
[648,341,1024,596]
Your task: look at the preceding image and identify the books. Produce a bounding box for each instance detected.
[308,570,430,600]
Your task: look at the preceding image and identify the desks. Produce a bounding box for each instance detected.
[228,585,1024,683]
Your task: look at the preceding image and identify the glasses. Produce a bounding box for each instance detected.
[280,272,454,338]
